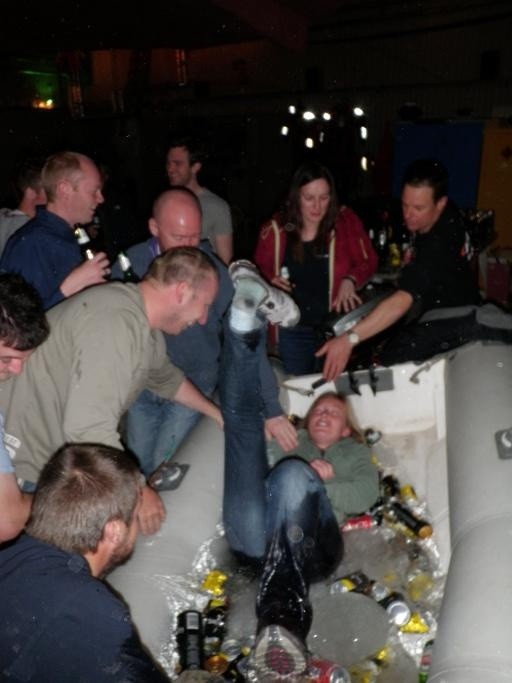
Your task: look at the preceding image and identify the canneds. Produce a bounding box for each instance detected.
[204,655,228,675]
[219,639,242,661]
[303,657,351,683]
[378,591,411,626]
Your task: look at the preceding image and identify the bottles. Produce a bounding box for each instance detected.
[369,229,390,267]
[113,241,141,284]
[280,262,310,327]
[73,222,112,279]
[366,429,397,468]
[400,222,412,260]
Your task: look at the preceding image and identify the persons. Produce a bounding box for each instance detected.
[1,442,174,683]
[1,151,112,312]
[314,159,479,383]
[164,140,232,264]
[1,246,224,536]
[217,257,379,681]
[84,223,101,239]
[253,164,378,376]
[112,186,299,480]
[0,167,47,261]
[0,264,50,545]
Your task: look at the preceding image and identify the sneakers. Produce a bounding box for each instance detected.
[227,259,301,331]
[254,625,309,683]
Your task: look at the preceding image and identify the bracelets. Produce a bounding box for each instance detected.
[341,276,357,288]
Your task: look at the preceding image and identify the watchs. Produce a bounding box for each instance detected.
[346,329,360,348]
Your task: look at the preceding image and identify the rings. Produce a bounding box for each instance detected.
[103,268,108,276]
